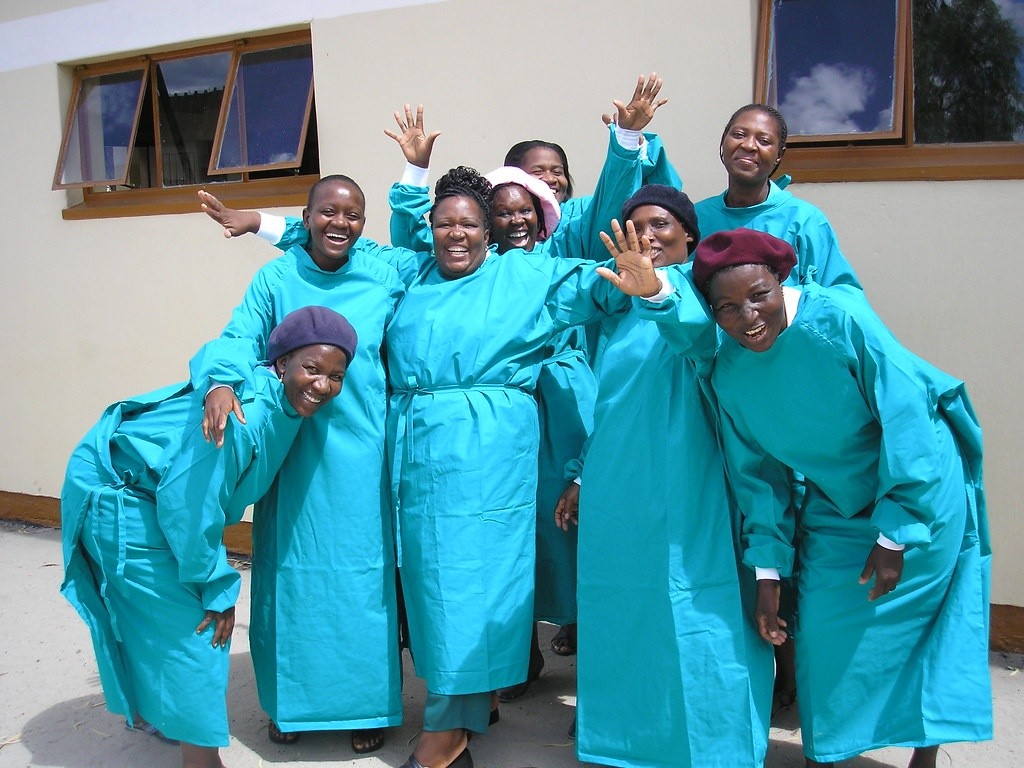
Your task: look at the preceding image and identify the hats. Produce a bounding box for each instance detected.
[484,165,561,241]
[622,184,701,256]
[692,229,798,295]
[266,305,358,367]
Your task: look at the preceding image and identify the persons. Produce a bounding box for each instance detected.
[691,228,992,768]
[555,186,777,768]
[503,110,683,656]
[59,305,357,768]
[188,173,407,754]
[692,104,862,720]
[198,165,633,768]
[385,72,667,740]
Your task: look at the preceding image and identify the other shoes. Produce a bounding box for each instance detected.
[268,717,299,744]
[351,728,385,754]
[496,665,545,701]
[770,683,796,722]
[550,622,577,656]
[400,743,473,768]
